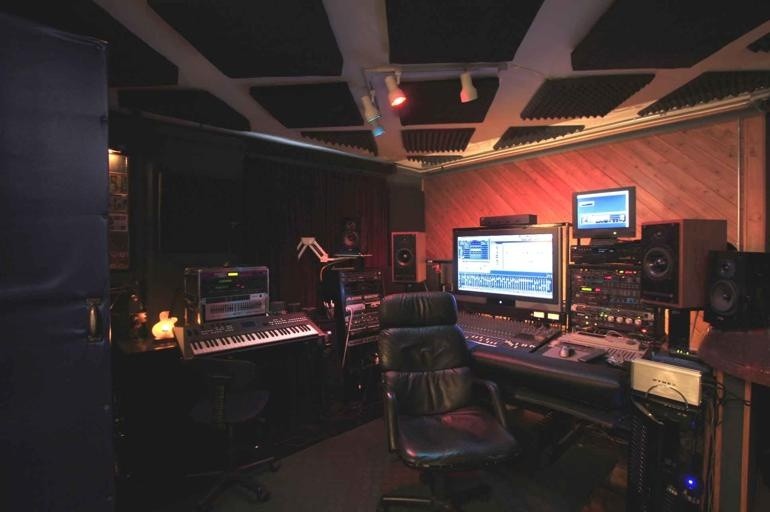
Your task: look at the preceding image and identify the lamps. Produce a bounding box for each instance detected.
[362,60,506,136]
[151,310,178,344]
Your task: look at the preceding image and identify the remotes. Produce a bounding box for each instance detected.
[578,350,607,362]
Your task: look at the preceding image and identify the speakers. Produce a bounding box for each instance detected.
[391,232,426,283]
[703,249,770,331]
[640,219,727,308]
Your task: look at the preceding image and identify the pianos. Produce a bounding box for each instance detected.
[184,311,326,363]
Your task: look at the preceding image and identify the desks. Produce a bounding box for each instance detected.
[697,330,769,511]
[115,337,176,356]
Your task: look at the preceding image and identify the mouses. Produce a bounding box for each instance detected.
[559,345,572,357]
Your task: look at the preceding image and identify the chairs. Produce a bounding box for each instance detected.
[183,358,281,512]
[377,291,519,512]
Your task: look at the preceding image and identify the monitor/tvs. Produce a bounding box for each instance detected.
[453,224,558,306]
[572,186,636,246]
